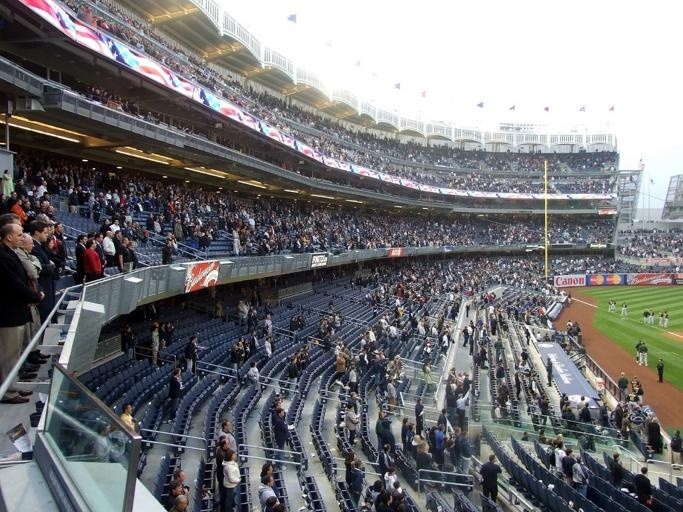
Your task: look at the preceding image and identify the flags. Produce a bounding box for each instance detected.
[395,83,401,89]
[510,104,515,111]
[421,91,426,97]
[477,101,483,107]
[580,106,585,112]
[544,106,548,111]
[287,13,296,23]
[609,105,614,111]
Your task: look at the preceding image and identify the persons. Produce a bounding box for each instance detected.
[1,0,682,511]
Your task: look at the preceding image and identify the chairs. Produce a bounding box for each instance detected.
[0,0,683,313]
[0,304,682,512]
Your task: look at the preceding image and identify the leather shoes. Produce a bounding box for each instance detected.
[0,350,51,404]
